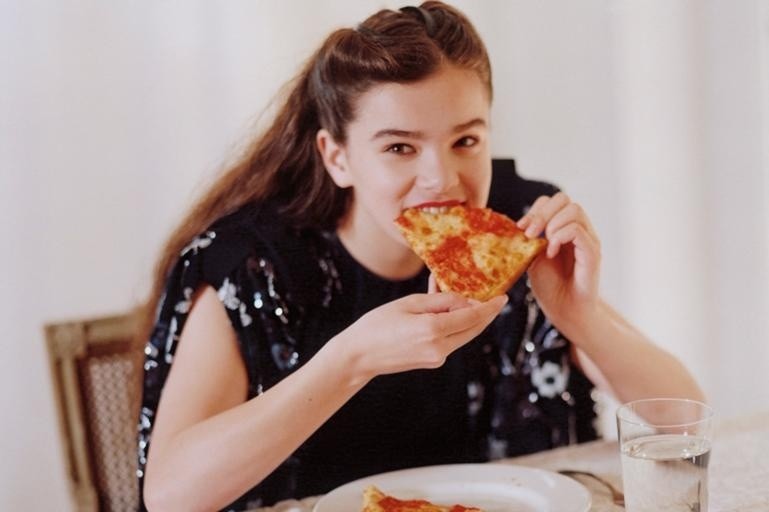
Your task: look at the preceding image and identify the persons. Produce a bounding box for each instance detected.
[129,1,704,512]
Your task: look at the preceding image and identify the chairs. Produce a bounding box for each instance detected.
[43,314,145,512]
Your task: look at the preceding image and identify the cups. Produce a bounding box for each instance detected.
[613,396,719,512]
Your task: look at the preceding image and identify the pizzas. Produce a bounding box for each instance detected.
[362,480,480,512]
[393,202,548,304]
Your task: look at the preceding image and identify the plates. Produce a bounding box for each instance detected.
[311,461,593,512]
